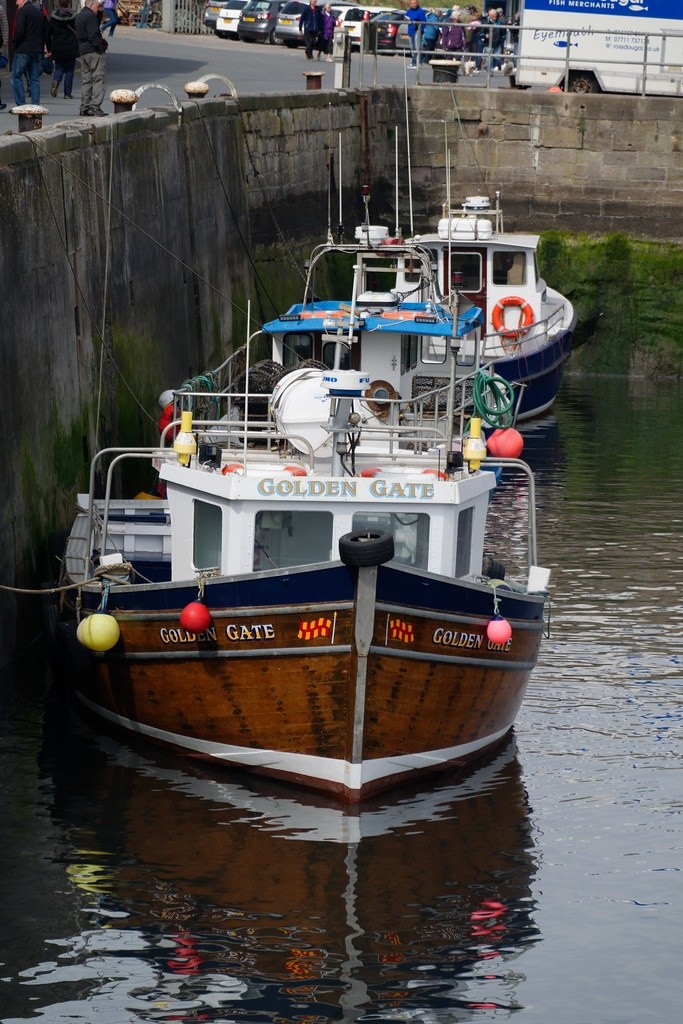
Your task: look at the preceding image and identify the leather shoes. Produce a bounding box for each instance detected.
[79,108,109,117]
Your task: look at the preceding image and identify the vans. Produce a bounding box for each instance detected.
[509,0,683,97]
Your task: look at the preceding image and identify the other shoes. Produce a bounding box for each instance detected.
[493,66,499,71]
[0,104,7,110]
[407,64,417,70]
[326,58,333,63]
[316,53,321,62]
[50,81,59,97]
[64,94,73,98]
[474,68,480,74]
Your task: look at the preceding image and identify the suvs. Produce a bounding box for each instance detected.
[203,0,452,56]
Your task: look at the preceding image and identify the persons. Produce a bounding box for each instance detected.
[0,0,118,117]
[405,0,520,76]
[299,0,336,62]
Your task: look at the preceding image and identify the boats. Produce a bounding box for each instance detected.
[34,726,538,1018]
[50,123,552,800]
[349,48,577,422]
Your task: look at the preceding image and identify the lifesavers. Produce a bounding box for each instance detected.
[159,400,182,438]
[492,296,533,339]
[338,527,394,567]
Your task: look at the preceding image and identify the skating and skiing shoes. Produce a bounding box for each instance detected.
[95,39,108,54]
